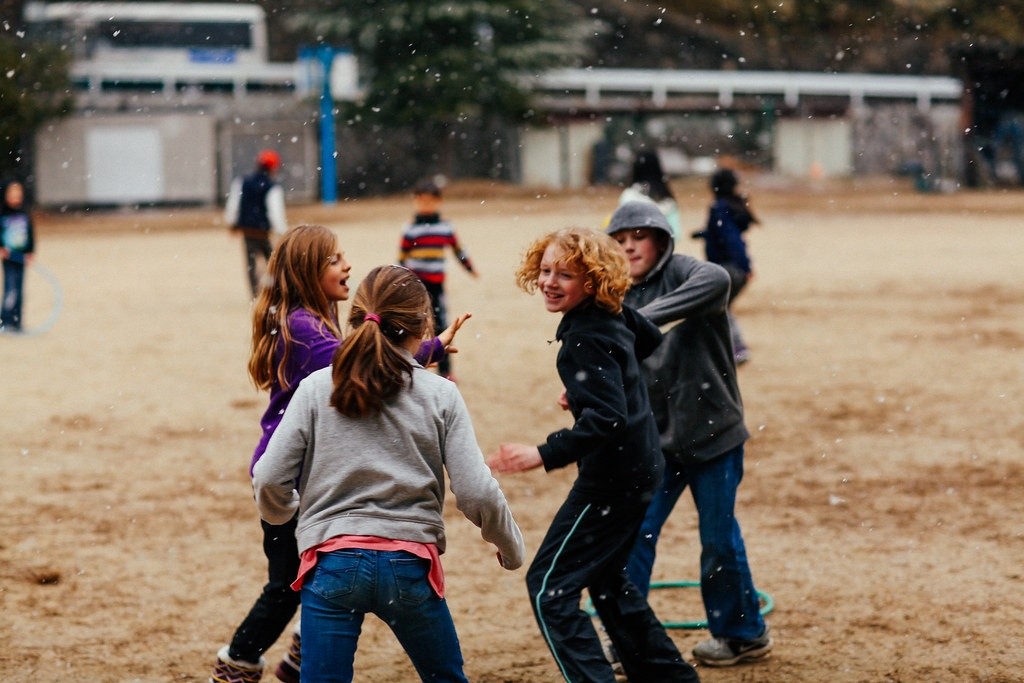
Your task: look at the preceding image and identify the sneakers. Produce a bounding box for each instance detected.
[691,622,773,665]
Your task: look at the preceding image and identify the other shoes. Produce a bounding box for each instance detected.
[736,344,749,366]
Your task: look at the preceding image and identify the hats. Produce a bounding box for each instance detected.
[632,151,662,180]
[712,169,736,189]
[257,150,280,171]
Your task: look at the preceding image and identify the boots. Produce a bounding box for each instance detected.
[208,645,266,683]
[275,620,300,683]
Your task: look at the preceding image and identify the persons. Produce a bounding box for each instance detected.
[398,181,480,384]
[619,143,760,367]
[605,199,774,666]
[252,264,526,683]
[0,178,35,334]
[221,148,290,298]
[485,226,701,683]
[209,225,472,683]
